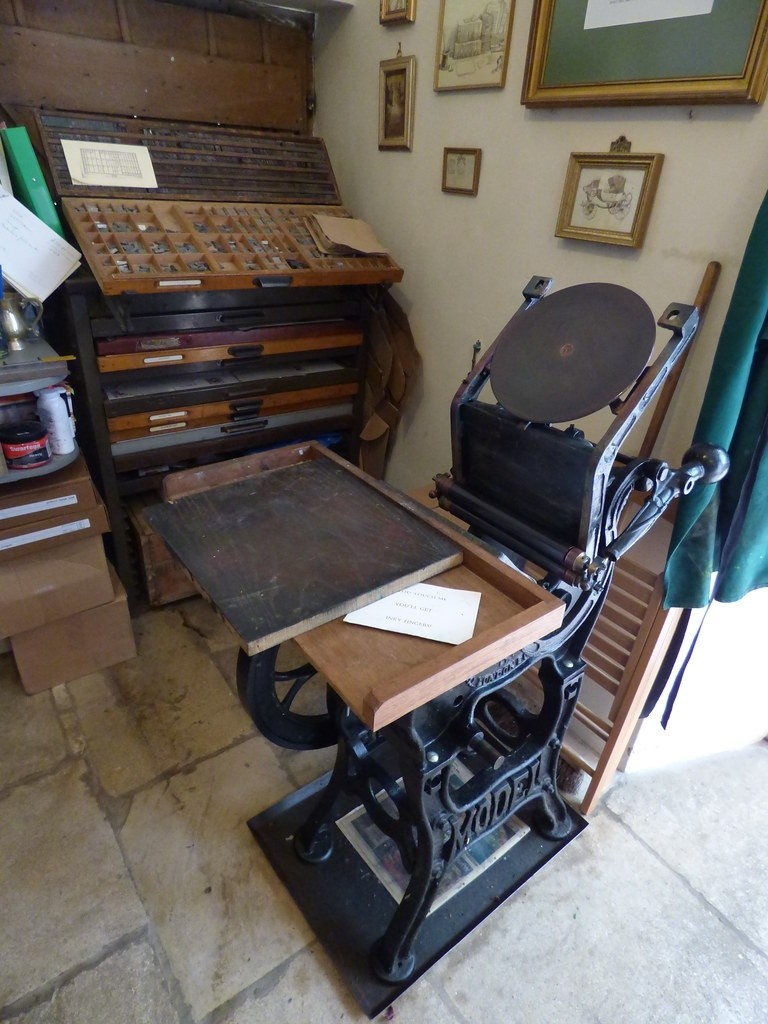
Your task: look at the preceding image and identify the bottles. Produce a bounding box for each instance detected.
[37,389,75,456]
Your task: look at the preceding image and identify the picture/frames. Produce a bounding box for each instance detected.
[376,55,413,152]
[379,0,417,26]
[519,0,768,110]
[441,146,483,196]
[431,1,515,92]
[553,136,666,248]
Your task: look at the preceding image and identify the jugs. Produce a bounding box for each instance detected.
[0,292,43,352]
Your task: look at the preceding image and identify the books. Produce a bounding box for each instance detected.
[0,185,81,308]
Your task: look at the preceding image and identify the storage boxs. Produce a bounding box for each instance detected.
[1,450,111,563]
[0,530,112,639]
[8,554,138,695]
